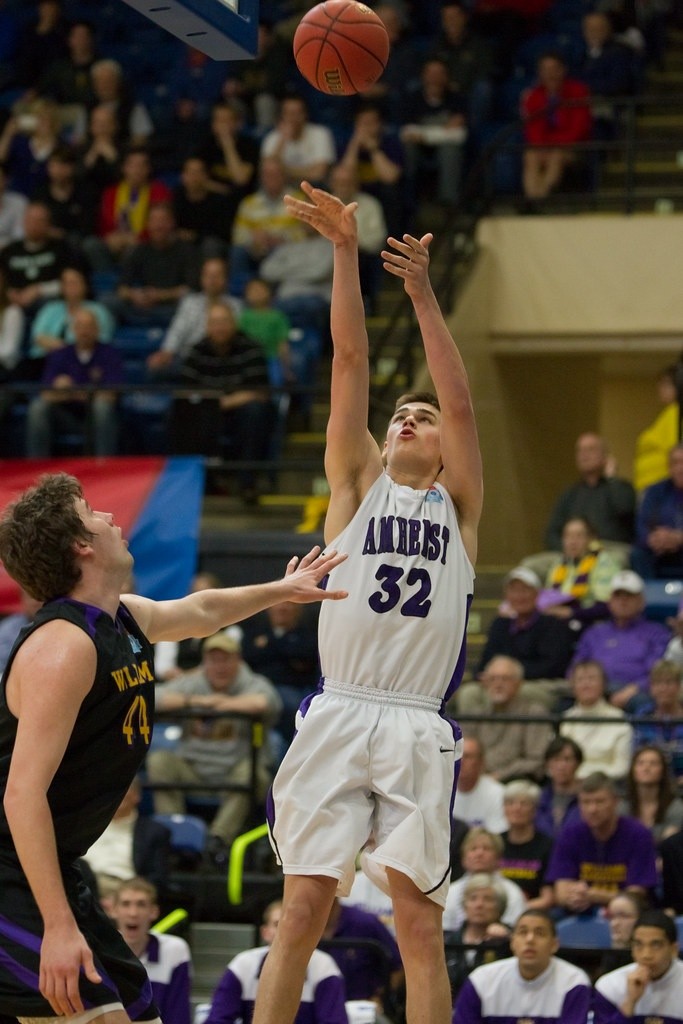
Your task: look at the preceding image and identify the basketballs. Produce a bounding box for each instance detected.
[292,0,392,98]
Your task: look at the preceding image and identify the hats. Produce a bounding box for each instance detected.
[610,569,644,595]
[203,630,239,654]
[508,566,541,591]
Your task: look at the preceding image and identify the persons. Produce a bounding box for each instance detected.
[248,179,485,1023]
[443,352,683,1024]
[0,574,408,1024]
[0,0,645,498]
[0,471,348,1024]
